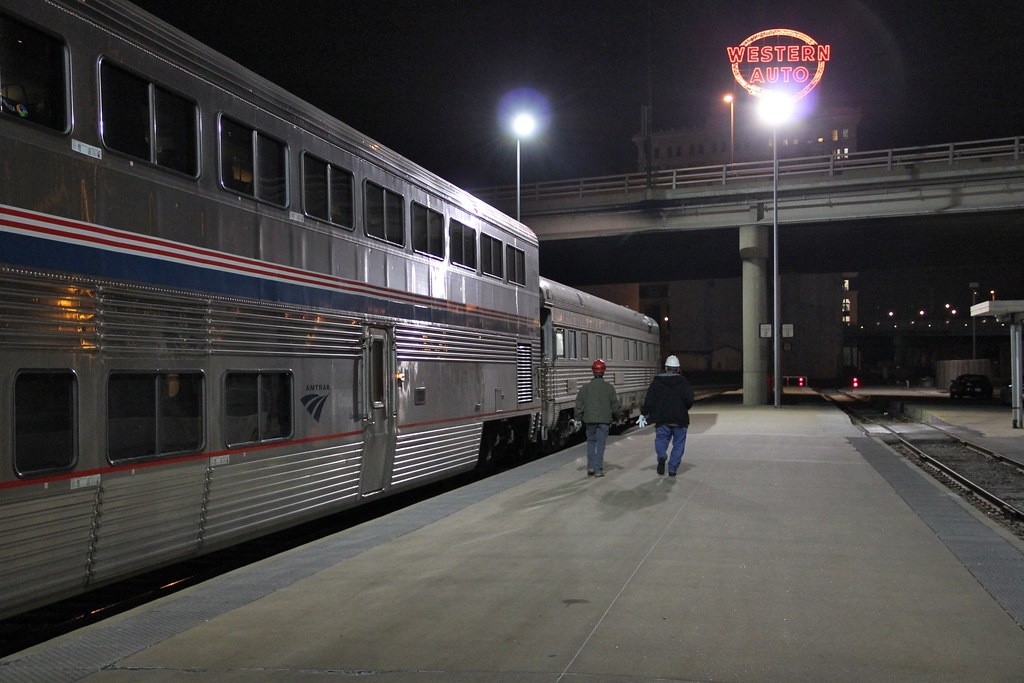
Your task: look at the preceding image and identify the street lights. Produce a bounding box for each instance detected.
[721,92,735,164]
[513,114,535,222]
[751,93,800,410]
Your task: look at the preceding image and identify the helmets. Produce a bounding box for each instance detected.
[665,355,681,367]
[592,359,607,373]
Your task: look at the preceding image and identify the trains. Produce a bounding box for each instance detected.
[1,1,661,638]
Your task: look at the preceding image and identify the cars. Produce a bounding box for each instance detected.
[999,376,1024,405]
[949,372,994,404]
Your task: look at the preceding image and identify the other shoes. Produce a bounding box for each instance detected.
[587,468,595,475]
[657,457,666,475]
[669,471,676,476]
[594,471,605,477]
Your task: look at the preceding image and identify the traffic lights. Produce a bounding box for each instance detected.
[798,377,805,386]
[853,377,858,389]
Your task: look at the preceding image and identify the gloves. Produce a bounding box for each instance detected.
[636,415,648,428]
[573,420,582,432]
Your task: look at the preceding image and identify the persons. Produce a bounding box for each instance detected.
[573,359,619,477]
[636,355,694,476]
